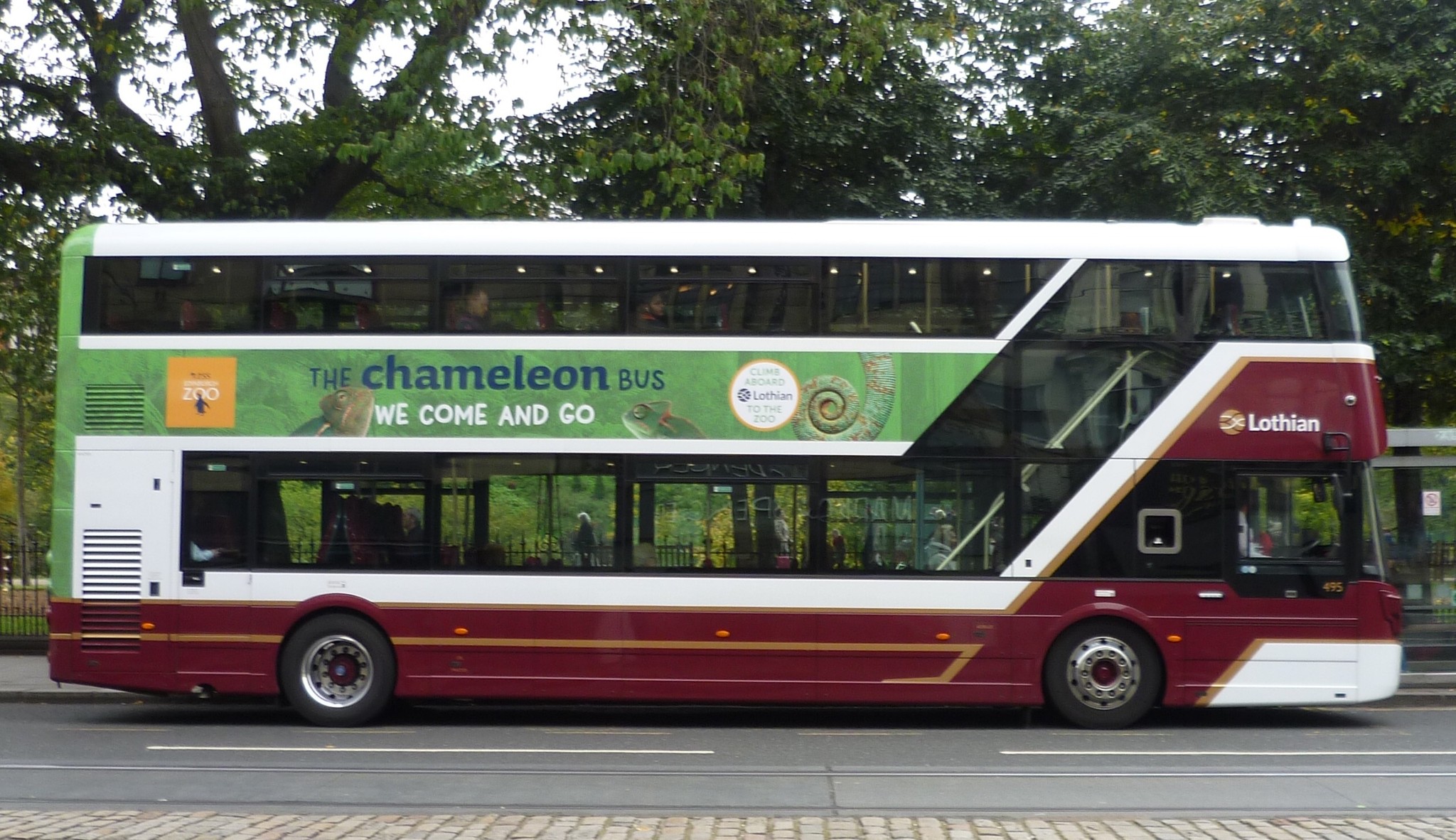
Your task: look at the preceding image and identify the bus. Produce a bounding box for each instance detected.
[43,216,1412,729]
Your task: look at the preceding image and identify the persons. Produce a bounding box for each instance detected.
[576,511,595,565]
[925,506,963,570]
[456,283,490,333]
[1239,490,1270,558]
[775,497,912,569]
[626,542,666,573]
[402,507,422,569]
[637,289,682,332]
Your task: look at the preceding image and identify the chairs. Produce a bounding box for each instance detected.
[537,300,557,331]
[890,539,914,571]
[716,303,731,334]
[1119,308,1149,333]
[284,308,299,332]
[1210,305,1241,336]
[354,303,384,333]
[264,301,286,332]
[179,301,204,329]
[619,296,637,334]
[192,477,567,571]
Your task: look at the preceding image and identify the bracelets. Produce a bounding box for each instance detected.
[1333,543,1339,546]
